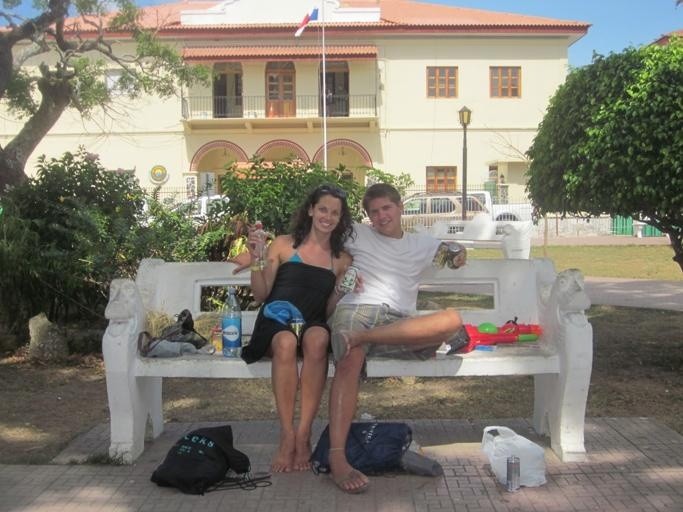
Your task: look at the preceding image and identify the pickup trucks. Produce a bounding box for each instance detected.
[462,190,534,234]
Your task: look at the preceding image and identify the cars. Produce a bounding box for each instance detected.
[142,194,230,230]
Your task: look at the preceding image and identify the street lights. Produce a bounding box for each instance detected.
[456,104,472,222]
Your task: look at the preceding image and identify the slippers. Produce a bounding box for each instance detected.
[328,468,370,493]
[330,330,350,361]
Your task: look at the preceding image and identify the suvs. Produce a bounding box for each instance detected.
[360,192,489,233]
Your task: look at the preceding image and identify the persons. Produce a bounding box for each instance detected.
[241,186,364,473]
[227,184,467,494]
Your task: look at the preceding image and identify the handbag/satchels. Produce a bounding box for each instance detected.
[151,425,250,496]
[309,422,411,470]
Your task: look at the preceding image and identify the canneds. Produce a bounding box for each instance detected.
[447,244,461,268]
[507,456,519,491]
[340,265,360,294]
[290,318,306,347]
[433,242,451,267]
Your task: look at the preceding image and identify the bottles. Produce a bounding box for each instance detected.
[249,220,267,272]
[212,287,242,357]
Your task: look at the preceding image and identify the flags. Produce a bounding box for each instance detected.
[295,3,319,37]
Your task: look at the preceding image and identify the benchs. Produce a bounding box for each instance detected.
[102,258,595,465]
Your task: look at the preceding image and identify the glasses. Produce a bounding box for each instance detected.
[319,185,346,197]
